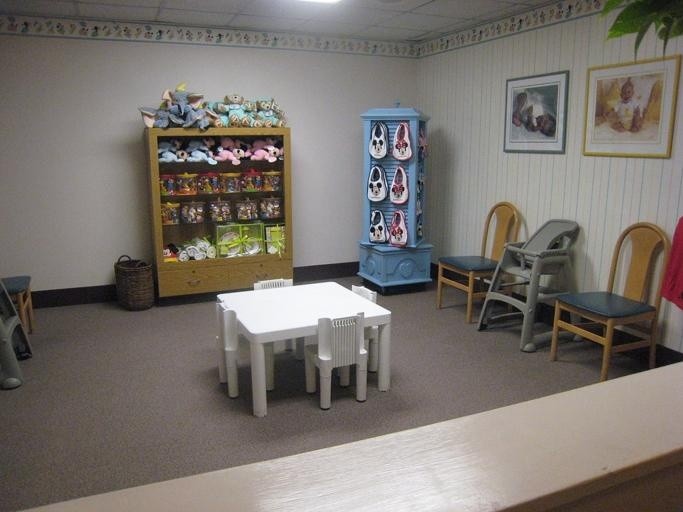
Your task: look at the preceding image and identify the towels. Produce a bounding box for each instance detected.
[176,237,216,262]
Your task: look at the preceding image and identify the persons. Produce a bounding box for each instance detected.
[606,78,642,133]
[512,92,556,137]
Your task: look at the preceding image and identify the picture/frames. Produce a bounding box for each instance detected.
[503,71,569,154]
[265,224,286,254]
[581,53,682,159]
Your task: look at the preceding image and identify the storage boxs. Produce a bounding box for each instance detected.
[215,224,263,258]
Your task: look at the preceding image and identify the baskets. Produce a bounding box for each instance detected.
[114,255,153,311]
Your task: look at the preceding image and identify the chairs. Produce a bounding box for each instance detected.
[215,278,378,411]
[438,202,521,324]
[549,222,672,383]
[476,219,578,353]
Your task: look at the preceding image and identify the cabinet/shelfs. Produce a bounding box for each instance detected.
[146,127,295,308]
[356,98,436,294]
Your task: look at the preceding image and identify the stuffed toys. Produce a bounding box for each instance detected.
[139,81,287,224]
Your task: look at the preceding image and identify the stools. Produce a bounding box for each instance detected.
[0,276,35,336]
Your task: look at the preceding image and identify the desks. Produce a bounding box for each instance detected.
[217,283,393,419]
